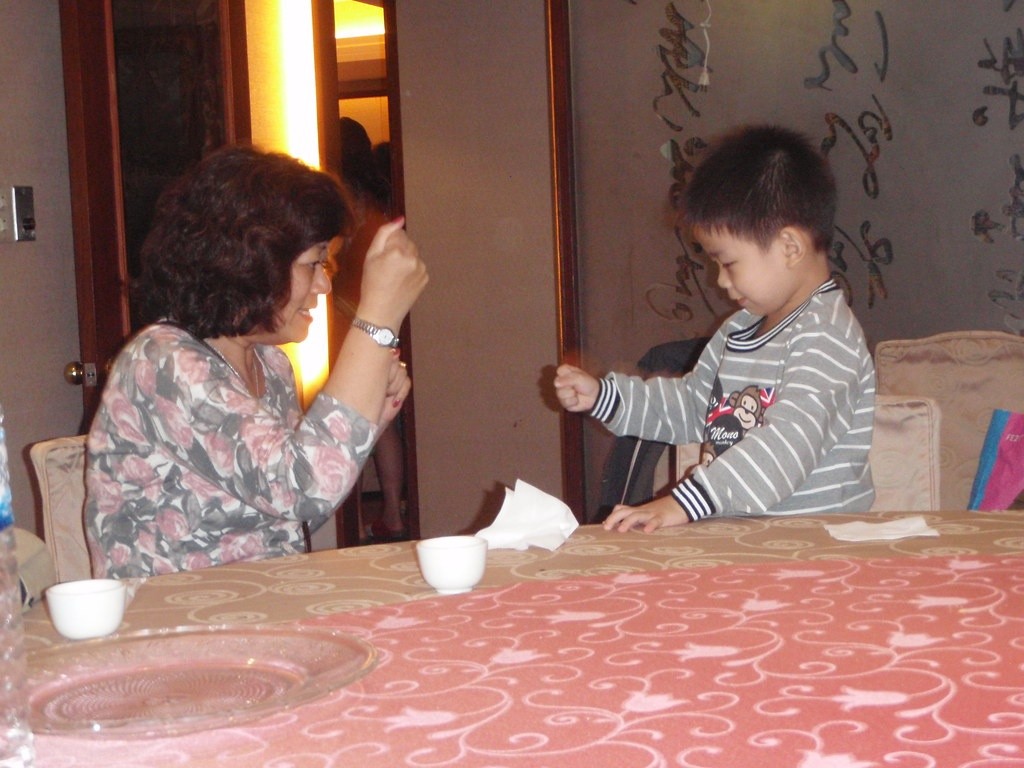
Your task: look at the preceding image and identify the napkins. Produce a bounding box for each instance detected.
[820,515,941,543]
[472,480,579,553]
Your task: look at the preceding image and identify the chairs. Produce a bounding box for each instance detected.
[661,328,1024,511]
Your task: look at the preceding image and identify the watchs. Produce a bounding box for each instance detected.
[351,318,398,348]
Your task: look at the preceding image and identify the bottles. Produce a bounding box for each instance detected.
[0,407,36,768]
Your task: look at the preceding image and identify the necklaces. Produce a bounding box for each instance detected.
[204,339,258,398]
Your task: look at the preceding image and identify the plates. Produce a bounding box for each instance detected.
[24,624,378,739]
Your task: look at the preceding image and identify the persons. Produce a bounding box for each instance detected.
[338,117,404,541]
[82,147,429,580]
[554,127,875,534]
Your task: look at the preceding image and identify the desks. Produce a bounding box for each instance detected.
[0,508,1024,768]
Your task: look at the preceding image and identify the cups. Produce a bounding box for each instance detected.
[414,536,486,595]
[45,578,125,640]
